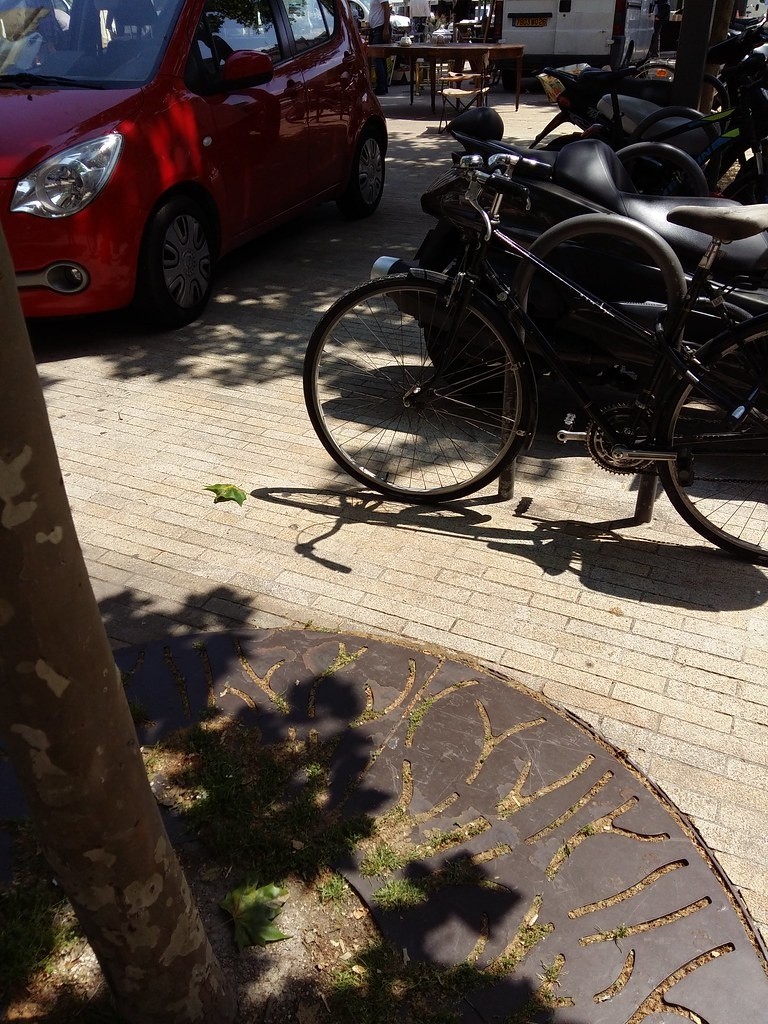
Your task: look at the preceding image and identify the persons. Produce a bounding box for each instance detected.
[409,0,458,43]
[368,0,392,96]
[653,0,670,22]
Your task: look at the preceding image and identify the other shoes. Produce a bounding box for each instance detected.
[373,87,388,95]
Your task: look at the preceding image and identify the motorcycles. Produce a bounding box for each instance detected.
[539,13,768,173]
[371,101,767,418]
[525,9,768,154]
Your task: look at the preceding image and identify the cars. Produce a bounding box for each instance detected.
[459,5,495,26]
[347,0,411,30]
[0,0,391,336]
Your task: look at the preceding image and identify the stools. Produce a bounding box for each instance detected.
[416,57,491,134]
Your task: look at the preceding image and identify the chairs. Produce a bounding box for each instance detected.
[106,0,161,79]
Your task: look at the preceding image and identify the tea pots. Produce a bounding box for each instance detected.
[400,32,412,47]
[430,24,453,47]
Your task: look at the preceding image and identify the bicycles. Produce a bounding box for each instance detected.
[303,149,768,566]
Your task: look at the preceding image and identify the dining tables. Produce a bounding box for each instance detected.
[364,42,526,114]
